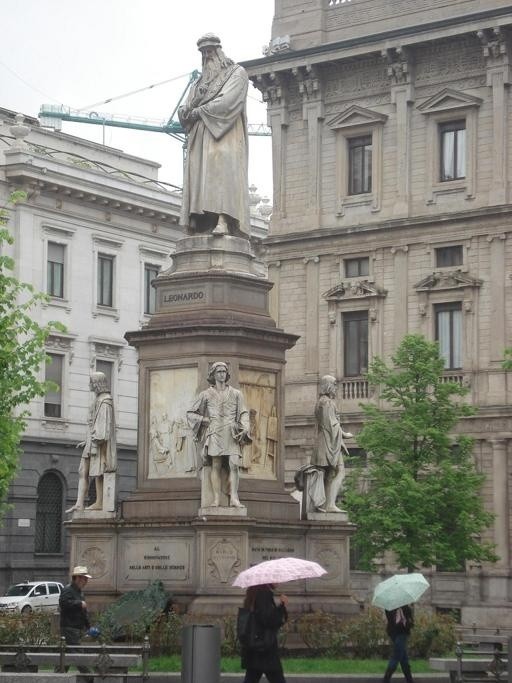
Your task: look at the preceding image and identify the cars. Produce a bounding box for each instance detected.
[0,580,65,616]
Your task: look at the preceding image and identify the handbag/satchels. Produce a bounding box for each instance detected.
[236,606,279,653]
[395,609,411,631]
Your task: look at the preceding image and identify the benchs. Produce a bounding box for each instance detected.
[428,653,510,683]
[0,646,141,683]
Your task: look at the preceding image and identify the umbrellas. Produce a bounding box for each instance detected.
[371,571,430,611]
[232,557,328,590]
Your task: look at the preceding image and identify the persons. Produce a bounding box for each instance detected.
[178,32,253,240]
[149,416,169,465]
[381,604,415,682]
[52,564,100,683]
[159,411,178,464]
[304,373,354,513]
[62,371,119,514]
[236,583,290,683]
[184,362,255,511]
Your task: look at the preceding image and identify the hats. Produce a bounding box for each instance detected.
[71,565,93,579]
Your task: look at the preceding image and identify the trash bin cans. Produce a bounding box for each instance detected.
[181,624,221,683]
